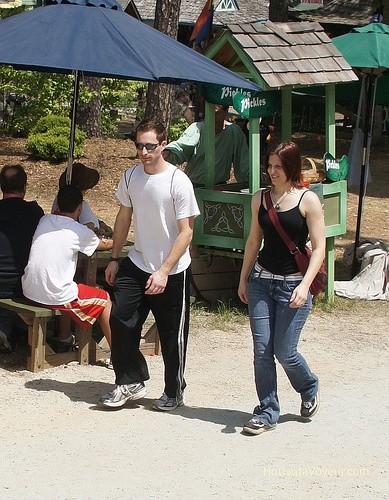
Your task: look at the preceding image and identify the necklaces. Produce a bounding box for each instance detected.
[271,185,296,210]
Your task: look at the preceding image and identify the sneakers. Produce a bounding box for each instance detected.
[152,390,183,411]
[102,379,147,406]
[301,390,318,419]
[243,418,275,435]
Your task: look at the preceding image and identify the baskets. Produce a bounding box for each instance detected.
[301,157,323,183]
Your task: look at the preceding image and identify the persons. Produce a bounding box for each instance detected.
[51,162,100,233]
[0,165,45,354]
[236,141,327,435]
[162,94,250,185]
[98,120,200,411]
[21,185,114,371]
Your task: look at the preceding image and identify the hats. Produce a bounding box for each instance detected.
[58,163,99,191]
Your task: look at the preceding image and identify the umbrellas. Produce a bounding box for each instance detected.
[0,0,262,188]
[330,17,389,279]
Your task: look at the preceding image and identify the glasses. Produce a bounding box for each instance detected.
[135,140,160,150]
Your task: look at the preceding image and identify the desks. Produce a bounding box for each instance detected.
[77,242,163,365]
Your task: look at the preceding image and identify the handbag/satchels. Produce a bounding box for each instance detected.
[289,247,327,295]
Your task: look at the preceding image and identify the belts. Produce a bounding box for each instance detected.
[253,262,303,282]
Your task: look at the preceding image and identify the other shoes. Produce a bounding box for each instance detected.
[59,335,77,352]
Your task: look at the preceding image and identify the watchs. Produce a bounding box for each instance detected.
[109,256,119,262]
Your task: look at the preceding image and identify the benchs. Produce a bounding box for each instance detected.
[1,297,79,373]
